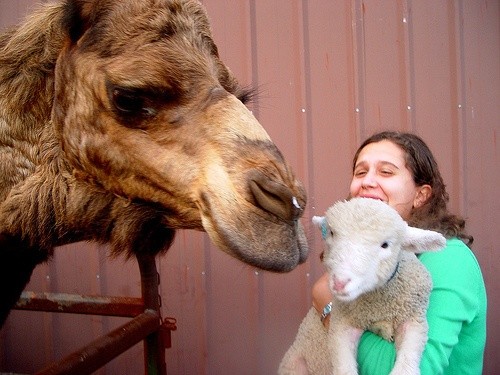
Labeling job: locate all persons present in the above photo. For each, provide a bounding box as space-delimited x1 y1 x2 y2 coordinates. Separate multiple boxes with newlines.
306 132 488 375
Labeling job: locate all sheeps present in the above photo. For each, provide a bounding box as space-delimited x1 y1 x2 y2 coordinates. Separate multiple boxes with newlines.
273 196 448 375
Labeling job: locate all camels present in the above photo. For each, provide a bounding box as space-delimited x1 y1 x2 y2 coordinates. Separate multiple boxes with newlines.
0 0 309 330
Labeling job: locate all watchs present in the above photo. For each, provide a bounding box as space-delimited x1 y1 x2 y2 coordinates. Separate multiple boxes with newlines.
318 300 332 326
